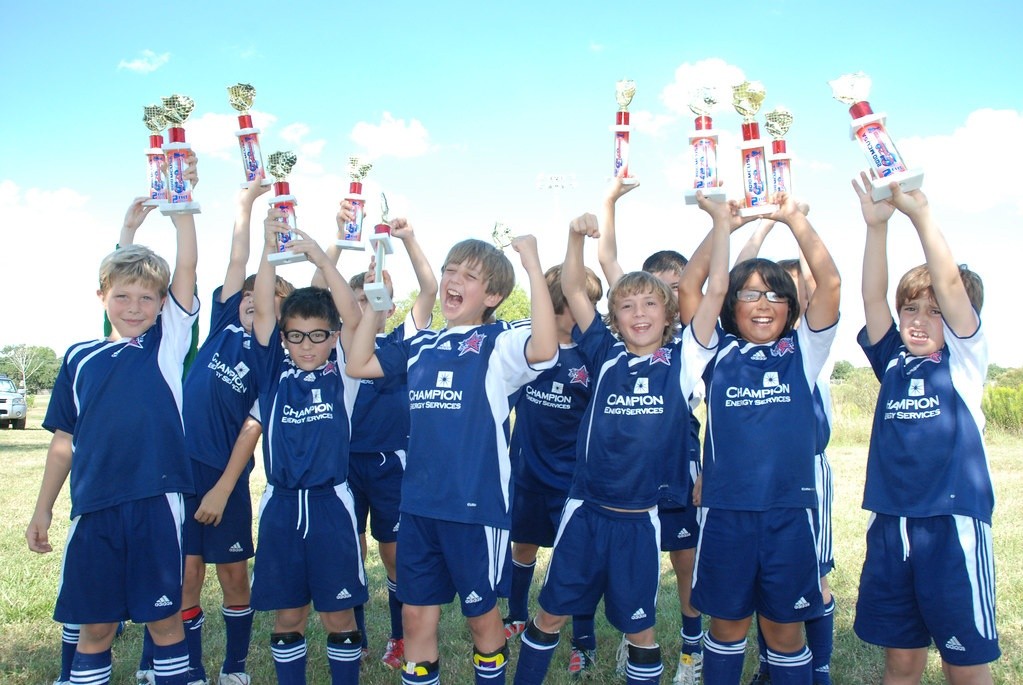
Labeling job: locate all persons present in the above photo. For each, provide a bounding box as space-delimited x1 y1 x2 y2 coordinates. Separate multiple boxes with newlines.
25 167 840 685
850 168 1001 685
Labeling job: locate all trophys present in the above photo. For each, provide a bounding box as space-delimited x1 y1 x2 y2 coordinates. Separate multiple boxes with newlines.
614 79 636 185
225 83 275 189
489 221 517 251
266 151 309 266
827 72 924 202
142 104 169 206
336 157 373 251
159 95 203 216
684 85 726 206
762 110 794 208
363 192 394 312
730 80 777 217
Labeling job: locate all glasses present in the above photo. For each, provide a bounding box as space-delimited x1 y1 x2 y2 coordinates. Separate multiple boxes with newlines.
736 289 789 303
283 329 335 344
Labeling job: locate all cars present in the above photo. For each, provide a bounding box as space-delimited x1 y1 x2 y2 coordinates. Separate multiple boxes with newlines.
18 385 25 396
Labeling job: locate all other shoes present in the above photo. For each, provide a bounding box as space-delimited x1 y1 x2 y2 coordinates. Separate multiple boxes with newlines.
749 673 769 685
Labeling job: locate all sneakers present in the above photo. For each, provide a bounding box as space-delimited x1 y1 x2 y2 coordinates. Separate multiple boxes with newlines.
188 678 210 685
570 638 596 681
673 649 703 685
135 670 155 685
217 666 250 685
615 634 629 681
501 615 529 641
381 638 405 671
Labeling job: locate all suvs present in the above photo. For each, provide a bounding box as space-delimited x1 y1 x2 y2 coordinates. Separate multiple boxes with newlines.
0 377 26 428
